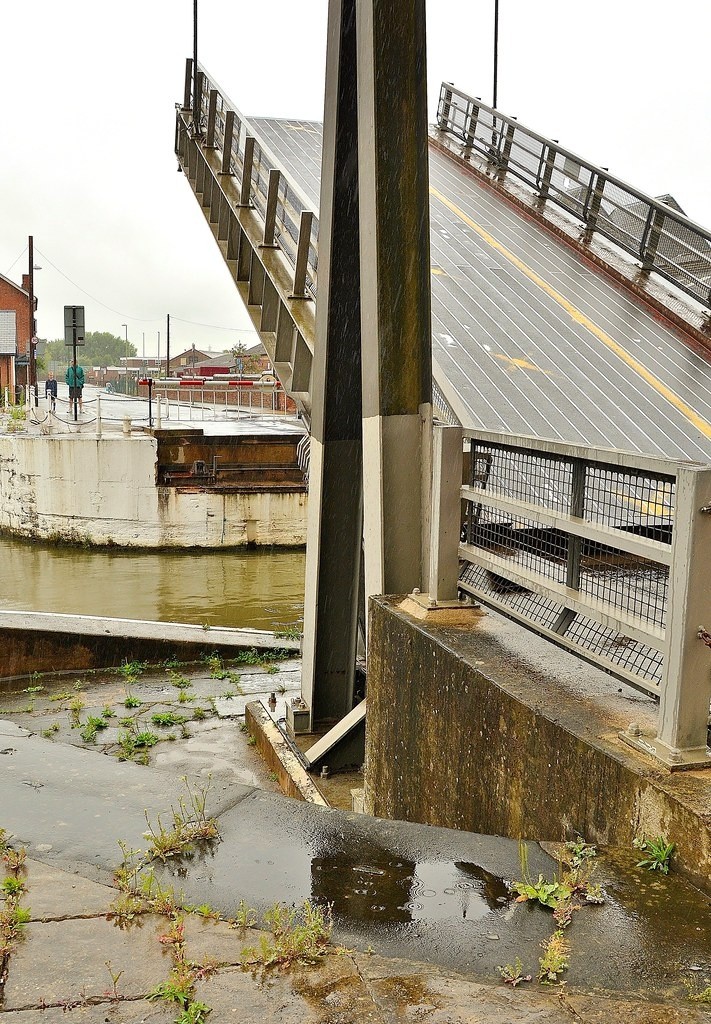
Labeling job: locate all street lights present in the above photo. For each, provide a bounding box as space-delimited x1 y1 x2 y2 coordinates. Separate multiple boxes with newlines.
122 324 128 395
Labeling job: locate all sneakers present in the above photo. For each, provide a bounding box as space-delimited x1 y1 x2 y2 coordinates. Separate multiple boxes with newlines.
80 409 85 413
67 409 73 413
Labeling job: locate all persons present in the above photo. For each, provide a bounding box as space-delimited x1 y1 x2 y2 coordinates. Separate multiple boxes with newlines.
65 357 86 413
45 371 57 413
106 381 117 394
